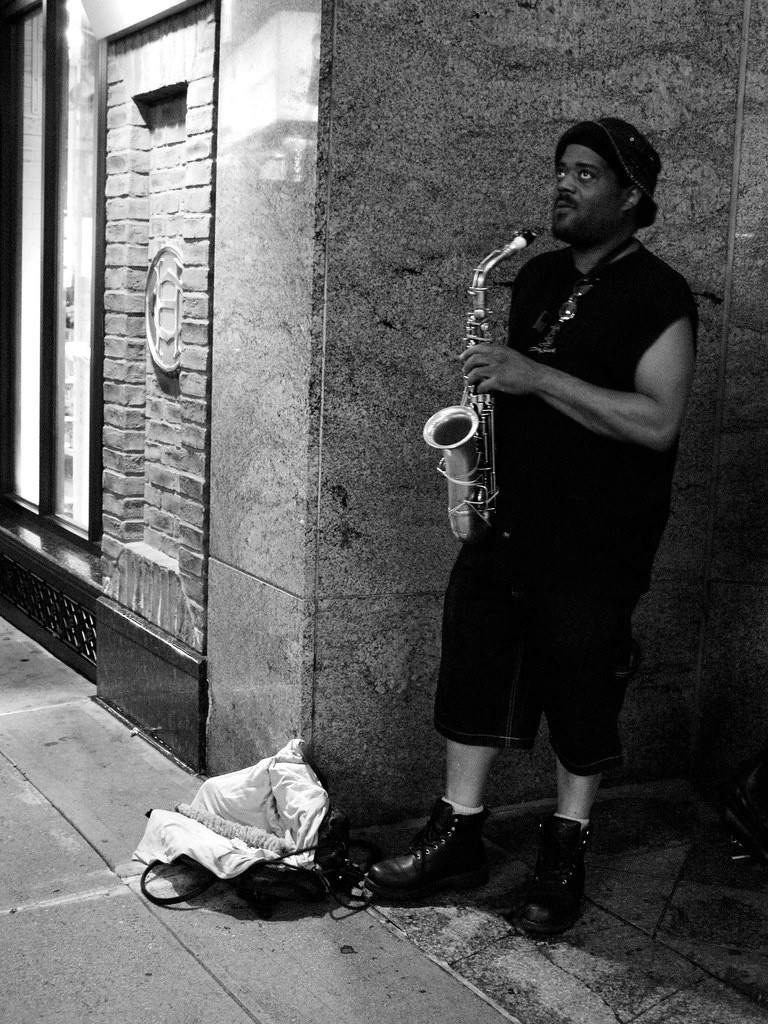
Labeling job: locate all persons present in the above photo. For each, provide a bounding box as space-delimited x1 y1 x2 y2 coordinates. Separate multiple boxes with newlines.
364 119 699 935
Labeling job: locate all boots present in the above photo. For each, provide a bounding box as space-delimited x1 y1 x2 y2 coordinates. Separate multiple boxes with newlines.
521 813 592 935
365 797 494 902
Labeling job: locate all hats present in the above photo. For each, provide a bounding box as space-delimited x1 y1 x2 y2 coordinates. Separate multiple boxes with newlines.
555 116 660 228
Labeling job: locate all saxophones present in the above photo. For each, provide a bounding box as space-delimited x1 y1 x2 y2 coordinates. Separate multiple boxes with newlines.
420 227 560 544
